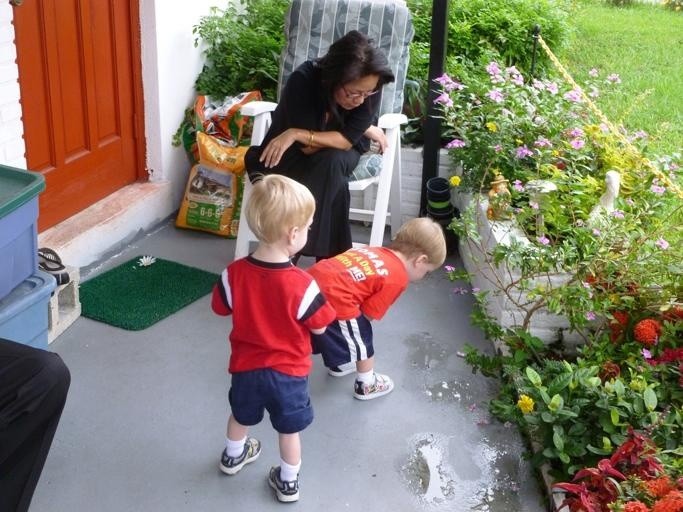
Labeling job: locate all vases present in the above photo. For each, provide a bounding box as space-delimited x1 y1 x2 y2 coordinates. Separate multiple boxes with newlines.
458 168 614 357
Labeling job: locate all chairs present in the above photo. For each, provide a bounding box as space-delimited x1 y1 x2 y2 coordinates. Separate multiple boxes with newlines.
233 0 415 261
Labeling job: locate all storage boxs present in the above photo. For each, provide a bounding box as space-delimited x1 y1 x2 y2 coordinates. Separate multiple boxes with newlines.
0 164 47 299
0 271 57 351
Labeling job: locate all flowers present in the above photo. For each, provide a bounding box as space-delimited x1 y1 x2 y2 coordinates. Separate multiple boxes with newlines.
428 59 683 256
137 254 157 267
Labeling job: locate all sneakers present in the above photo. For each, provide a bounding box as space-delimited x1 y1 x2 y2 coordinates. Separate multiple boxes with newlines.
328 362 358 376
220 437 262 475
356 372 394 400
268 464 300 502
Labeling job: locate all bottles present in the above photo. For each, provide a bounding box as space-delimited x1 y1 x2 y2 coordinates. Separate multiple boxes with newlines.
425 177 450 211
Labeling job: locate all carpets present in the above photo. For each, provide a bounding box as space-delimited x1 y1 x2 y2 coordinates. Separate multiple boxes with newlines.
79 255 223 331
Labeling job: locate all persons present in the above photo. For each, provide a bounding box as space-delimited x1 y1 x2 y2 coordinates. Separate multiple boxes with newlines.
302 216 449 403
208 172 337 504
244 29 398 266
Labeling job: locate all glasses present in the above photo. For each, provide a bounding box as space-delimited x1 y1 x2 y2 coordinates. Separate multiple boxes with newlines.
340 84 380 99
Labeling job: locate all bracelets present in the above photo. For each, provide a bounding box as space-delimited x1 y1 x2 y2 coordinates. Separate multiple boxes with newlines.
305 130 314 147
